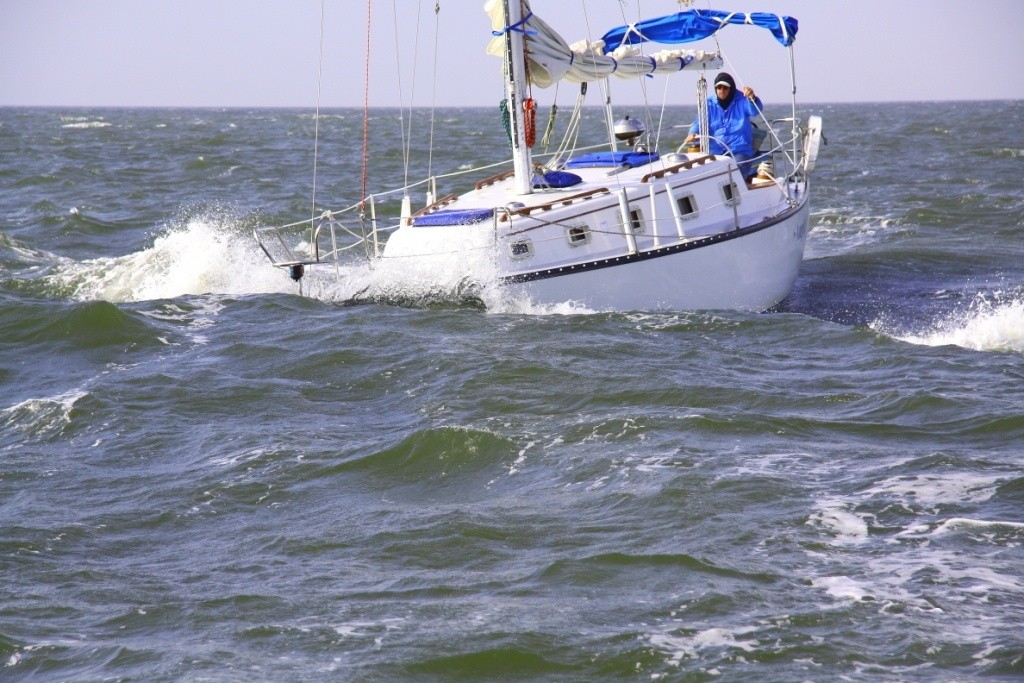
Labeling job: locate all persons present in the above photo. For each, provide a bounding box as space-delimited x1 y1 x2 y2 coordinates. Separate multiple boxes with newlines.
685 72 763 186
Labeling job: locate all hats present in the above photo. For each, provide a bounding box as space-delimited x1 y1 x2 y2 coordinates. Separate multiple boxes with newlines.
714 80 731 88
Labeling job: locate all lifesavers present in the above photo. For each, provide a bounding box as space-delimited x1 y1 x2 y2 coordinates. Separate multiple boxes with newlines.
803 114 823 172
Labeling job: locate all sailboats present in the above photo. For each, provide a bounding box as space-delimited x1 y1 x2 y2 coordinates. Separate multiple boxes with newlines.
253 0 831 316
